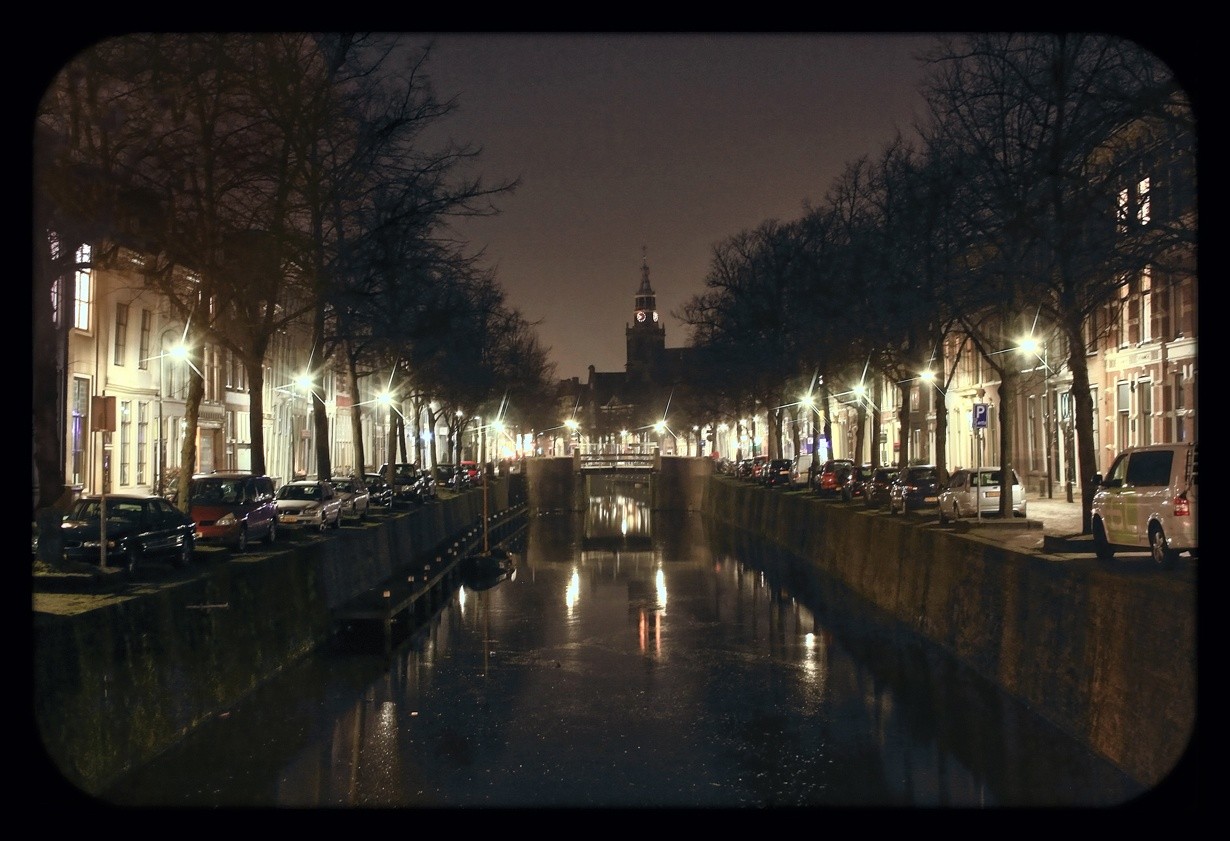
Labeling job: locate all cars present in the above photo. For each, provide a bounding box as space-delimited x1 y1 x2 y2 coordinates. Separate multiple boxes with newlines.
840 465 873 505
330 474 371 522
429 462 472 493
812 464 825 494
364 473 394 511
416 467 438 501
459 459 482 486
863 465 899 510
27 492 196 580
817 459 857 498
274 479 343 534
889 464 951 518
733 455 793 489
936 465 1027 527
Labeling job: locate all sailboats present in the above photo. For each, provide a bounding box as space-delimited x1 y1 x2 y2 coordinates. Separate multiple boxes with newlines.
460 430 516 589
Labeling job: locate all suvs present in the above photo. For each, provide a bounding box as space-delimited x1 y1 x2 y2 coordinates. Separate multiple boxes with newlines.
378 463 427 506
172 470 279 552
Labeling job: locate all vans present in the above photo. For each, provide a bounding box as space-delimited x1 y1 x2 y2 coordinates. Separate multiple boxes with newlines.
1088 441 1201 573
788 452 820 492
